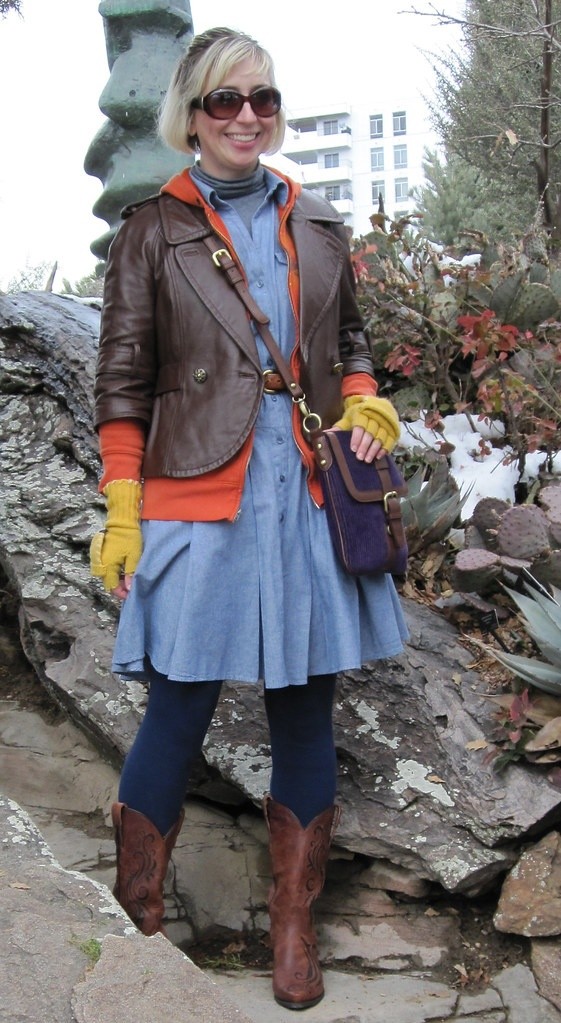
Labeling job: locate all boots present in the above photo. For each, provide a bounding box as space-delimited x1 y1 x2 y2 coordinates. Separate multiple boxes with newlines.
112 803 185 936
261 793 340 1010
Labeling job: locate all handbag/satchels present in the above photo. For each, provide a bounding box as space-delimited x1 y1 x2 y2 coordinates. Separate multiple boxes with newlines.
309 428 408 576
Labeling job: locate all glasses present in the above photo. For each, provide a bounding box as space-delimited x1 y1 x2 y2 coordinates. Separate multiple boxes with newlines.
191 86 282 120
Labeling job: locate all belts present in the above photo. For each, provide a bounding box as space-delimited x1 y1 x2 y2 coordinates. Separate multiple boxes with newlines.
261 369 287 395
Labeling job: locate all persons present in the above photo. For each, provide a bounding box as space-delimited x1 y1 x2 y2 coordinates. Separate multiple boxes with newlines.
88 26 400 1012
